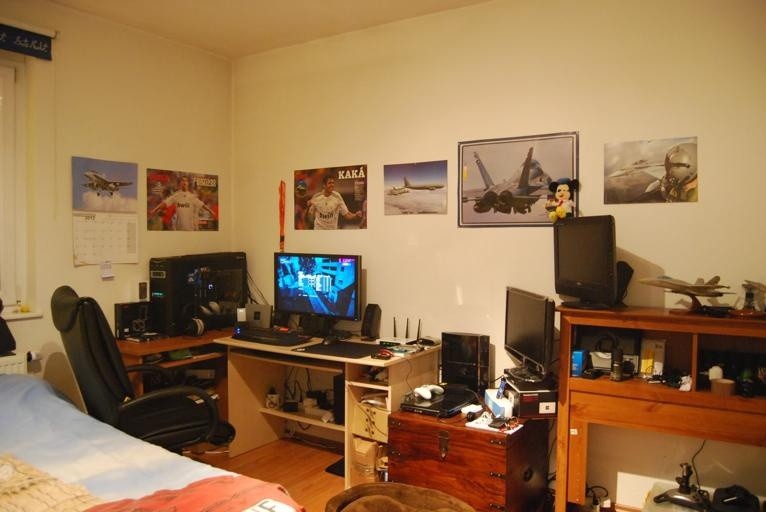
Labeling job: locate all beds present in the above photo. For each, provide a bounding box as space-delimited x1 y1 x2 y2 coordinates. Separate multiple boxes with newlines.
1 371 308 512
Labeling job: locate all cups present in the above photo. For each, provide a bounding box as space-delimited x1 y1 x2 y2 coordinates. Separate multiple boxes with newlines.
265 393 281 410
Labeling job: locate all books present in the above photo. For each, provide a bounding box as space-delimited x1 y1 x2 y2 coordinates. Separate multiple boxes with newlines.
389 345 419 355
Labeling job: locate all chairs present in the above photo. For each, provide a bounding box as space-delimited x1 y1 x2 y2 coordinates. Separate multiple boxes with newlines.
51 285 236 456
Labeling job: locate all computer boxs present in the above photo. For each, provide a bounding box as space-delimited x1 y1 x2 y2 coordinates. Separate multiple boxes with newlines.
149 251 247 336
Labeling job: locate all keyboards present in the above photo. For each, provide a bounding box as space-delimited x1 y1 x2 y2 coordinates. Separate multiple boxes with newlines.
232 328 307 346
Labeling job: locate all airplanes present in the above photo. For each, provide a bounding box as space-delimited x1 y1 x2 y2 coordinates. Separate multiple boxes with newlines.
610 158 666 179
386 186 409 196
461 144 555 218
391 174 445 193
77 171 133 198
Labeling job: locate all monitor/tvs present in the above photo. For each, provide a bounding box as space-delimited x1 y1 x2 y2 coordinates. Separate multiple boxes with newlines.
503 286 555 383
273 252 362 341
553 215 618 309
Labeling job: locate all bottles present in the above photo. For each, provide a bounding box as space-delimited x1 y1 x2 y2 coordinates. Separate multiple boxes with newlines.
744 293 754 310
611 362 622 382
739 366 753 396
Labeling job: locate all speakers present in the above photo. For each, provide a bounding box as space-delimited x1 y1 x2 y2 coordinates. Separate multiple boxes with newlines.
361 304 382 338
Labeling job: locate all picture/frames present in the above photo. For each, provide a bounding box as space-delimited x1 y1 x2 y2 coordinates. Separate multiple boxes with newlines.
457 131 578 228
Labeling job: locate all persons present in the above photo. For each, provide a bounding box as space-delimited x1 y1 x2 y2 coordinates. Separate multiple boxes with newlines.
660 142 697 202
295 169 367 230
147 174 217 231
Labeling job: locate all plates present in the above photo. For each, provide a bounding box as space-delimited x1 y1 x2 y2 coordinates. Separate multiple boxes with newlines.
728 309 765 318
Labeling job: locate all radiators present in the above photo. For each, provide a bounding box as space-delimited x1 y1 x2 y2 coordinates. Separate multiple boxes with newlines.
1 352 28 376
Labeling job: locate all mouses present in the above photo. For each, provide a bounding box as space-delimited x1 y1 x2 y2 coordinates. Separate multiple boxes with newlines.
371 349 392 359
323 336 339 346
